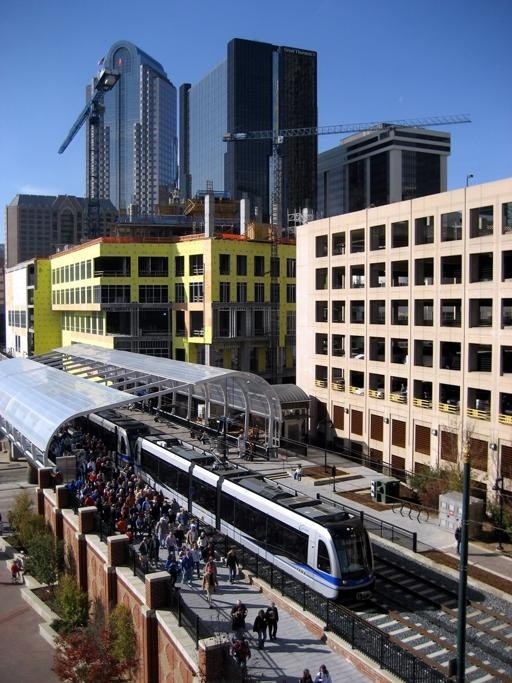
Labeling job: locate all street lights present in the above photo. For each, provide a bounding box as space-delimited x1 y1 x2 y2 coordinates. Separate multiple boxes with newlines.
316 419 333 473
493 475 504 551
217 417 227 462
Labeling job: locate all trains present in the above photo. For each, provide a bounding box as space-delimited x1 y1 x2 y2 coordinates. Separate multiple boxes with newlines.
67 409 376 601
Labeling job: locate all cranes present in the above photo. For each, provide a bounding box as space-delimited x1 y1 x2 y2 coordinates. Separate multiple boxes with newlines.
222 114 472 384
58 67 121 244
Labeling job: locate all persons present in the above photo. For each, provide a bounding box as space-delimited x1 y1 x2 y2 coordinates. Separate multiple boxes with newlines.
226 548 239 584
253 602 279 649
300 664 330 682
51 401 215 585
202 567 218 608
293 465 303 480
231 600 247 640
455 522 461 554
11 561 23 584
231 635 250 671
